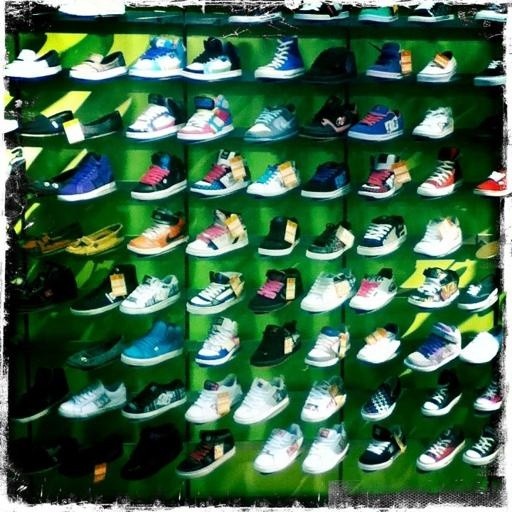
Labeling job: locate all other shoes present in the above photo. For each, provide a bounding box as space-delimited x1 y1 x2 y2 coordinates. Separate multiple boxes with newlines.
7 1 125 16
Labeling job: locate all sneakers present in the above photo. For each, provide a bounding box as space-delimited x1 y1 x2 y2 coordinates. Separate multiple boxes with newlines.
4 35 506 87
15 321 502 369
5 264 502 315
229 0 507 23
18 208 463 259
16 422 504 477
4 93 455 142
6 150 511 201
11 371 502 425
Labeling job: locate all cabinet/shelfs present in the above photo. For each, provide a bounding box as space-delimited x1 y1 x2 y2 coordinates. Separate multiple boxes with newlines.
4 18 506 495
